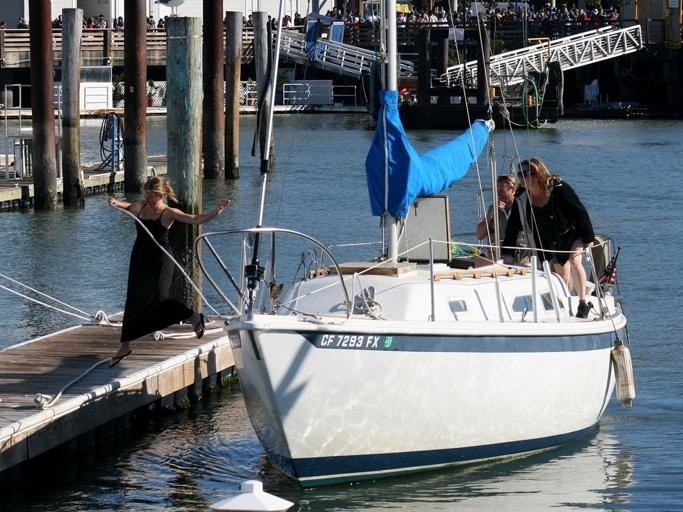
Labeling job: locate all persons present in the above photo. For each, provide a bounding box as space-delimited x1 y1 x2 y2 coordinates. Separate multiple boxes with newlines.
476 176 518 257
108 177 230 367
503 158 595 318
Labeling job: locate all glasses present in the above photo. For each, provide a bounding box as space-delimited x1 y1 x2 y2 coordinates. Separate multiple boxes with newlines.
517 171 530 179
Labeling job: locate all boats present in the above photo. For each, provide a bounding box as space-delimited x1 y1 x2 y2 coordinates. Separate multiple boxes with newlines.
398 69 548 131
191 0 627 490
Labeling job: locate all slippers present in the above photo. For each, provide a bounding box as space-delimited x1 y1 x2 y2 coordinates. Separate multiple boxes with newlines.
109 350 133 368
193 314 204 339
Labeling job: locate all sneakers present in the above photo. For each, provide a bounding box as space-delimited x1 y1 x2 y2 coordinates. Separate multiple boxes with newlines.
576 302 594 317
